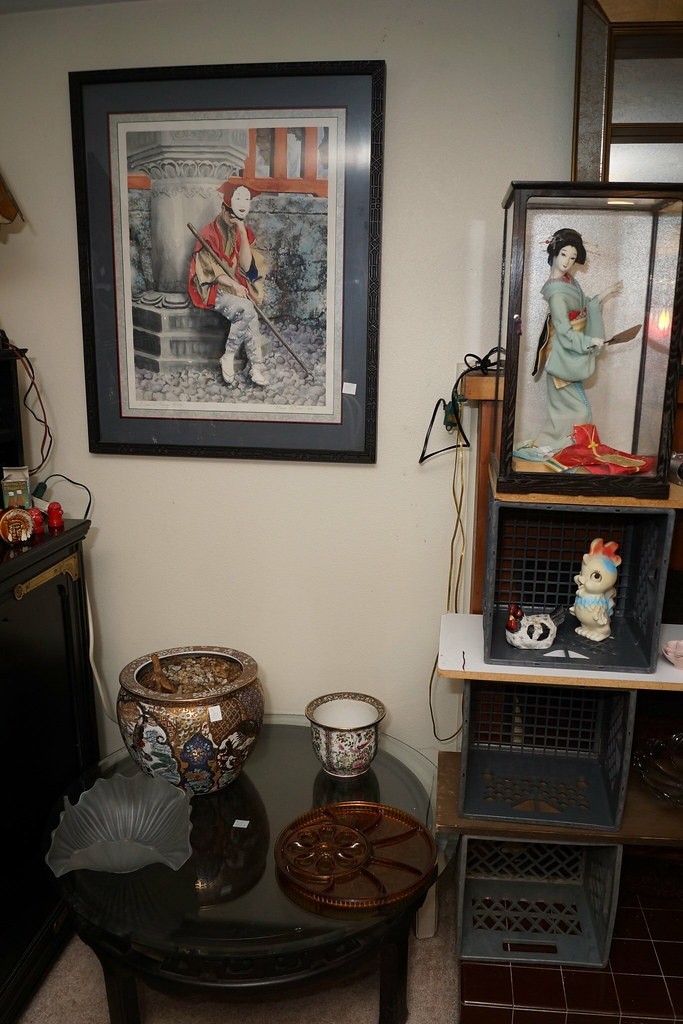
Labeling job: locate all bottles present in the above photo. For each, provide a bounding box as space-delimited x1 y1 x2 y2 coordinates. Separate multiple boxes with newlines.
184 774 271 908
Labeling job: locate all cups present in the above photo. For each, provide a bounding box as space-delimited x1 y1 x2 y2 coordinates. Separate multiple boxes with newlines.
305 692 387 778
310 769 382 808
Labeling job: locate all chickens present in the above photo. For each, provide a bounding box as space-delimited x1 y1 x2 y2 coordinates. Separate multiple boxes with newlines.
505 603 566 650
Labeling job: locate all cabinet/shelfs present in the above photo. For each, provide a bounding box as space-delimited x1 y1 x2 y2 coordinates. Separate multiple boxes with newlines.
0 350 100 1024
432 1 683 846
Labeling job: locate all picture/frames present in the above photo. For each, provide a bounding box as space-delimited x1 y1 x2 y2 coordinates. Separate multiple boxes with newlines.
68 60 387 463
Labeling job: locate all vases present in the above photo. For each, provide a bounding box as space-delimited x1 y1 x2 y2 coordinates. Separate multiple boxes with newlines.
305 692 384 778
116 645 267 797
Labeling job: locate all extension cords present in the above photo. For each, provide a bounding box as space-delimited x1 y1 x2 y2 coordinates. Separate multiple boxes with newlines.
31 494 52 515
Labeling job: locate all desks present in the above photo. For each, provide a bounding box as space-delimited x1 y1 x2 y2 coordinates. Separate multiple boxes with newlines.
61 712 459 1024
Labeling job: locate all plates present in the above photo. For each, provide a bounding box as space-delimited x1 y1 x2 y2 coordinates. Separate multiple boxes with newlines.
43 768 195 880
632 731 683 812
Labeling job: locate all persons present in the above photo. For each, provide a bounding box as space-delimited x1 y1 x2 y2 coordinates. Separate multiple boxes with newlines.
531 228 624 452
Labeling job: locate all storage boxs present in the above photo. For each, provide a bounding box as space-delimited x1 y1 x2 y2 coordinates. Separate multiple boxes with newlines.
483 484 675 675
460 679 637 830
456 832 624 970
2 466 32 511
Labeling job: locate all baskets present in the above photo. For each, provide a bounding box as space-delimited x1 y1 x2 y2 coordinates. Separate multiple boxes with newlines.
454 832 622 968
481 489 675 674
458 677 636 831
